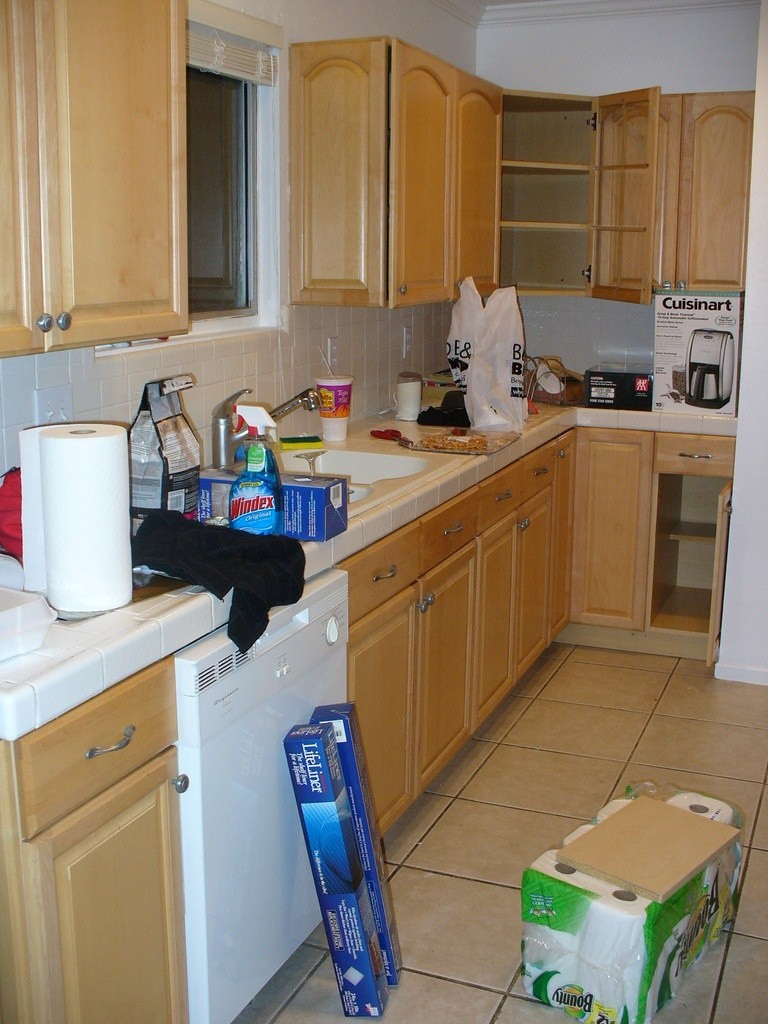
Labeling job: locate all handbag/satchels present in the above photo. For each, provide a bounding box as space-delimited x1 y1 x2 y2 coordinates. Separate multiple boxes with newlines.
523 352 585 406
446 275 528 433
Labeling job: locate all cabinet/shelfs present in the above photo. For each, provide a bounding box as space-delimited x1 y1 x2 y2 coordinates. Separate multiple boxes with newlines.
0 407 737 1024
0 0 189 358
292 36 758 308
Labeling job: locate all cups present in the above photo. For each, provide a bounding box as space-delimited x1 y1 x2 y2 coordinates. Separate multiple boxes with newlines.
314 374 355 442
394 371 422 421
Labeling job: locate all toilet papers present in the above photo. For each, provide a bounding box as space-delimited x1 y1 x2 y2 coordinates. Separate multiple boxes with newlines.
19 424 132 613
520 789 741 1024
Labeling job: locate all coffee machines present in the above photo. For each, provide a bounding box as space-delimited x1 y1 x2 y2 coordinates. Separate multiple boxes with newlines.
685 327 735 409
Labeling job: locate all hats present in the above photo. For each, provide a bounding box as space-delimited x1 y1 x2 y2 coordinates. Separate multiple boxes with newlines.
416 391 471 429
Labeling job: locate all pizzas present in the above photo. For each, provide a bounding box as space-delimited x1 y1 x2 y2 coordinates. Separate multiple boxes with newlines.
421 435 488 450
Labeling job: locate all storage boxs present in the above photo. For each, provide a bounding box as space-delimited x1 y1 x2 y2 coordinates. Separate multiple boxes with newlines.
654 290 742 420
198 467 347 542
284 723 389 1016
584 362 653 411
309 704 403 987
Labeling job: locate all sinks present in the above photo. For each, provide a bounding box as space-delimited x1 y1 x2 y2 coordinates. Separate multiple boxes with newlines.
349 484 373 506
229 447 428 485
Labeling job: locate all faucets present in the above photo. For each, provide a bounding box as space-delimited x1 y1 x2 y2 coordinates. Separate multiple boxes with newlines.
211 385 324 470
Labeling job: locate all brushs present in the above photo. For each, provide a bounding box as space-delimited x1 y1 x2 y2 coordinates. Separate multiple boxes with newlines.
538 356 584 382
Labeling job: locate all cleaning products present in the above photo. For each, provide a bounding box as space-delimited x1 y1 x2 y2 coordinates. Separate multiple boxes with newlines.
229 405 287 539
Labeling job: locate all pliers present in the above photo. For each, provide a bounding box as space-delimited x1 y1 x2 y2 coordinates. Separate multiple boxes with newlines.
371 429 414 448
